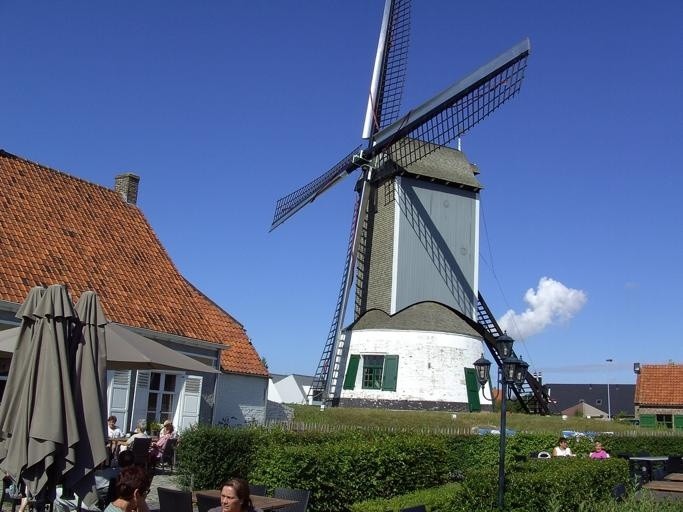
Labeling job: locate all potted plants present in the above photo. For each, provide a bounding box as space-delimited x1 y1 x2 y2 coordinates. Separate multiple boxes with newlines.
147 419 161 436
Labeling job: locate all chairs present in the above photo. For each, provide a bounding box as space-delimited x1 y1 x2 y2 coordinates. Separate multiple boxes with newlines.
2 476 21 512
154 438 177 475
125 437 153 475
248 485 267 496
157 488 193 512
196 493 221 512
270 487 310 512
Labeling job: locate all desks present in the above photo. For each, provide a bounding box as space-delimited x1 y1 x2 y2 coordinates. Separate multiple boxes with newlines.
196 488 300 512
101 434 159 468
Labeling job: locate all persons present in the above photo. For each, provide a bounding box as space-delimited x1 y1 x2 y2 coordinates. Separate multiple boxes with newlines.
107 416 178 468
552 437 572 456
590 439 607 459
207 478 264 512
103 465 149 512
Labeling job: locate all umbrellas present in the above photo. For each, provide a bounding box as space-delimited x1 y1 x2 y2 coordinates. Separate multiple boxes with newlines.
73 289 109 508
0 284 81 499
0 286 46 460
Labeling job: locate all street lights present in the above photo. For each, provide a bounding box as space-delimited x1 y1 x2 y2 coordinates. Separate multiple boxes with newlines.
471 329 529 508
